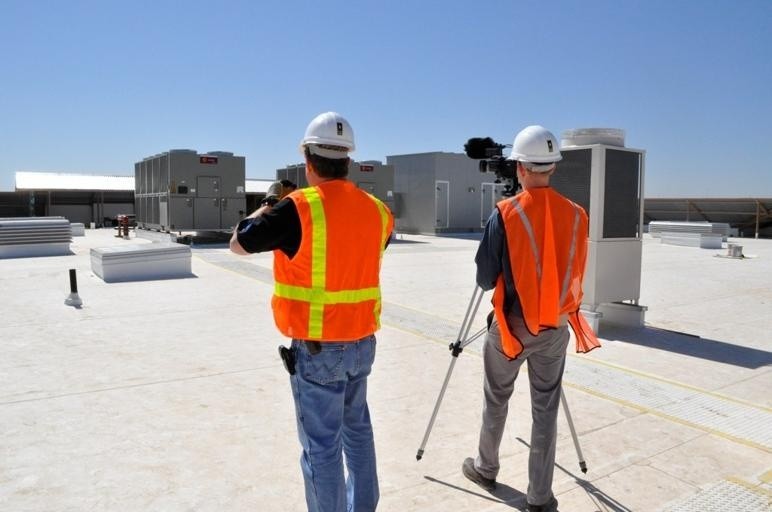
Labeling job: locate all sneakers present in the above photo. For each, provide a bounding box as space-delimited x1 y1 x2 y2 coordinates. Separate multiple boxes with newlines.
463 456 496 489
525 497 558 511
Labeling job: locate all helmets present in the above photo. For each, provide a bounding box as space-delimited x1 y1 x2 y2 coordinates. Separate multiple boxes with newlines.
505 126 563 163
299 110 354 154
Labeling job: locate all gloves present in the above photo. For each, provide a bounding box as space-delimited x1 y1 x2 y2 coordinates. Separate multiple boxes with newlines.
265 179 297 206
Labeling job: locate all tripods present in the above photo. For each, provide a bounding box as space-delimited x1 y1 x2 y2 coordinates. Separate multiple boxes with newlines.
414 193 589 473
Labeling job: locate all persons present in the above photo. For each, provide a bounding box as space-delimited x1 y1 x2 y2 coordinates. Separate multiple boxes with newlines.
458 124 600 512
228 110 395 512
